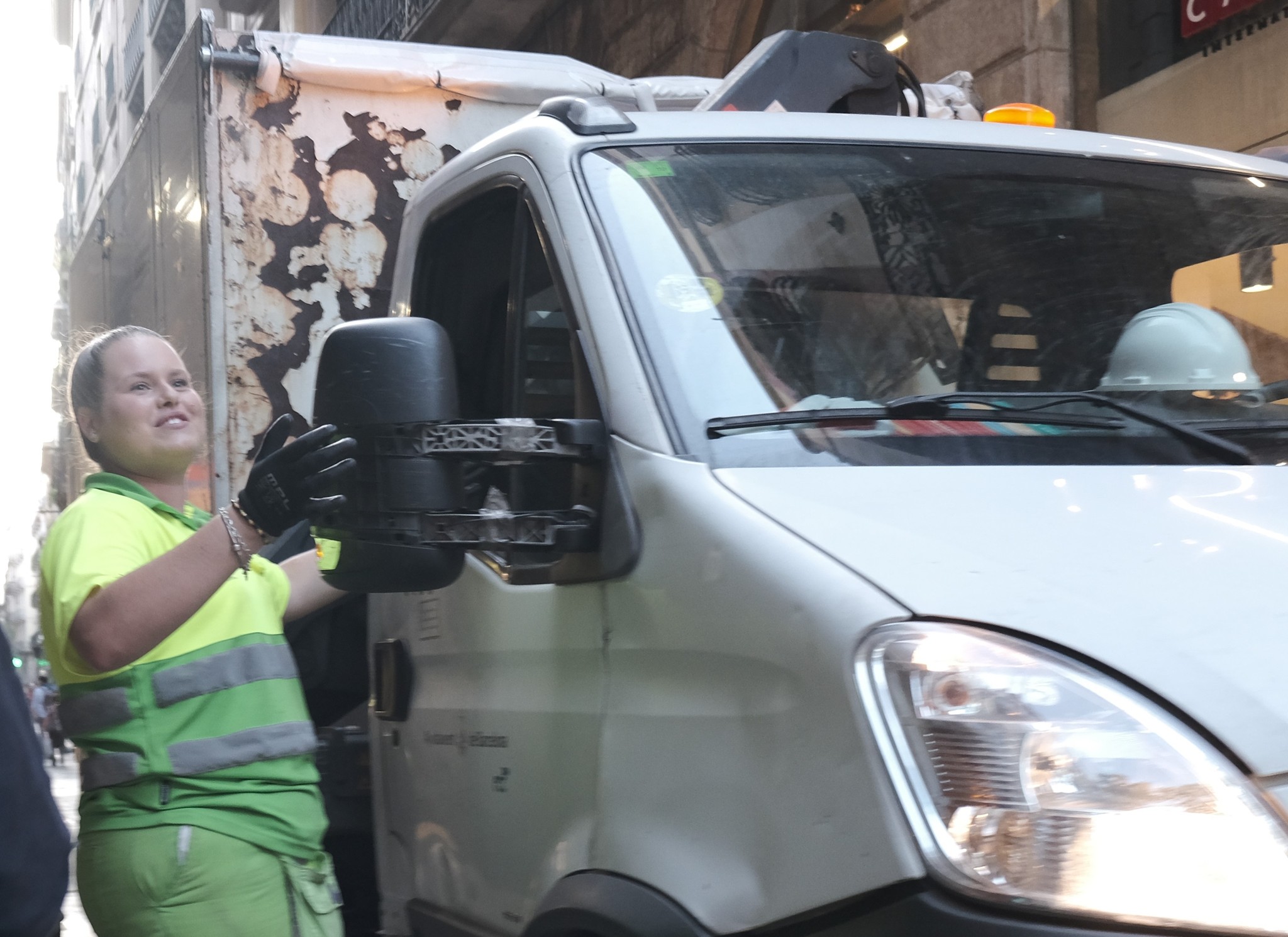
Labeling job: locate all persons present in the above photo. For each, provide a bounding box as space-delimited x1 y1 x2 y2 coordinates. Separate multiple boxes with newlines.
0 626 78 937
35 326 358 937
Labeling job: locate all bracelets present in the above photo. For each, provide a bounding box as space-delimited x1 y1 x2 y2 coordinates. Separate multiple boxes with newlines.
217 499 273 579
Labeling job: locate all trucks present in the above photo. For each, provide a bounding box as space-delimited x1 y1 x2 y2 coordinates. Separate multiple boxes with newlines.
49 4 1288 932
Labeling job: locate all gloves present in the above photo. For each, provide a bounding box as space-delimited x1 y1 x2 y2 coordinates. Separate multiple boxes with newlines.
238 413 357 538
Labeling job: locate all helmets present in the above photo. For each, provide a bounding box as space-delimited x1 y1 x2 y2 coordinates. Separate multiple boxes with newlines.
1093 303 1267 392
